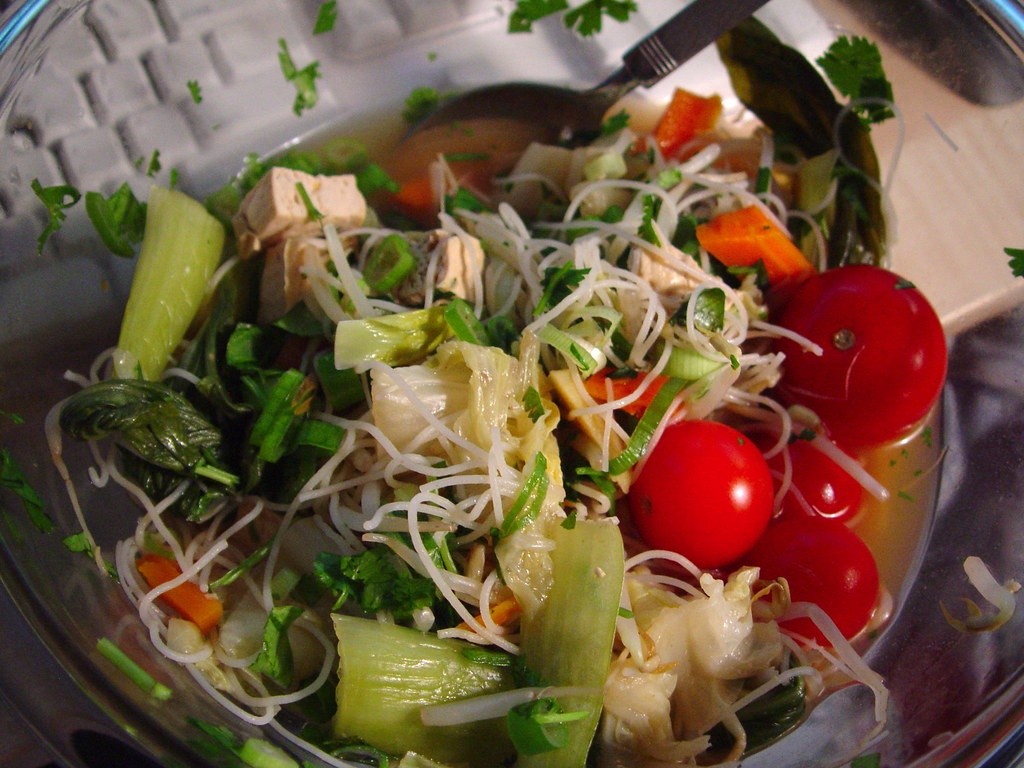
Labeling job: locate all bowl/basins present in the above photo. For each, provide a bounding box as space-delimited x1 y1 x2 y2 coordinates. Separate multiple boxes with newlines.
3 87 948 768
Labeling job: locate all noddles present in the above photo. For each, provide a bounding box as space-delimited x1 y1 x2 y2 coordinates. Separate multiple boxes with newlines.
73 90 948 766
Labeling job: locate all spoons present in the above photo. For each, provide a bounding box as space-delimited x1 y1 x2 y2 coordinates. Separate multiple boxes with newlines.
402 3 789 148
